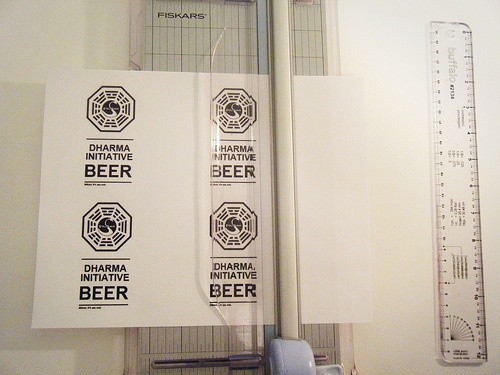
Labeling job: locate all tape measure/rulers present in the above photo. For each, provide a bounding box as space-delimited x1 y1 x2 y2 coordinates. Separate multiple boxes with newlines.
430 18 490 370
